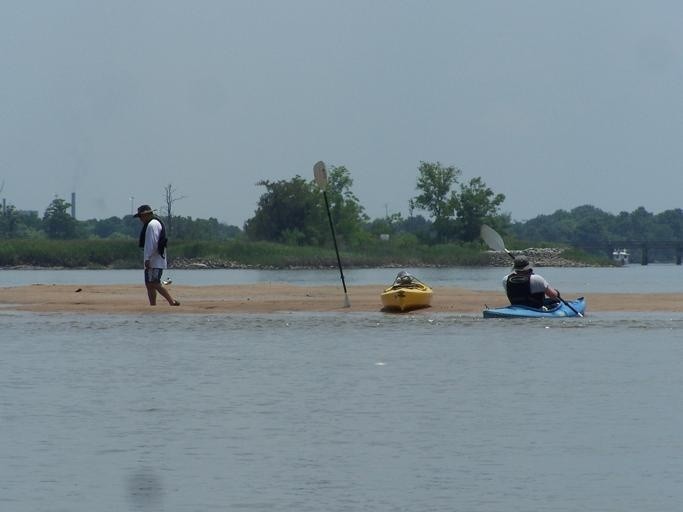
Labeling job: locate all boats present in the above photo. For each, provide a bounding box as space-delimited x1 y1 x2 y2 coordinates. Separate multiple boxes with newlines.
380 271 432 310
483 297 586 318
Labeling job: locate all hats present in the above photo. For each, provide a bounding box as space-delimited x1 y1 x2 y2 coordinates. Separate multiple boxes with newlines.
510 255 532 273
130 205 156 219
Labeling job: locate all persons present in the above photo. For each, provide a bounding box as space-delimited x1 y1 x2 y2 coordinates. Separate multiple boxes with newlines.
132 203 183 307
501 255 561 311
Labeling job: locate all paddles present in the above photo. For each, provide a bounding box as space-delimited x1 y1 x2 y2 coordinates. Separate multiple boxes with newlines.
313 161 349 308
480 224 583 318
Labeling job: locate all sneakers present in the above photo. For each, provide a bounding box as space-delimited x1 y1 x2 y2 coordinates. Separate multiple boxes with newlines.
170 300 179 306
